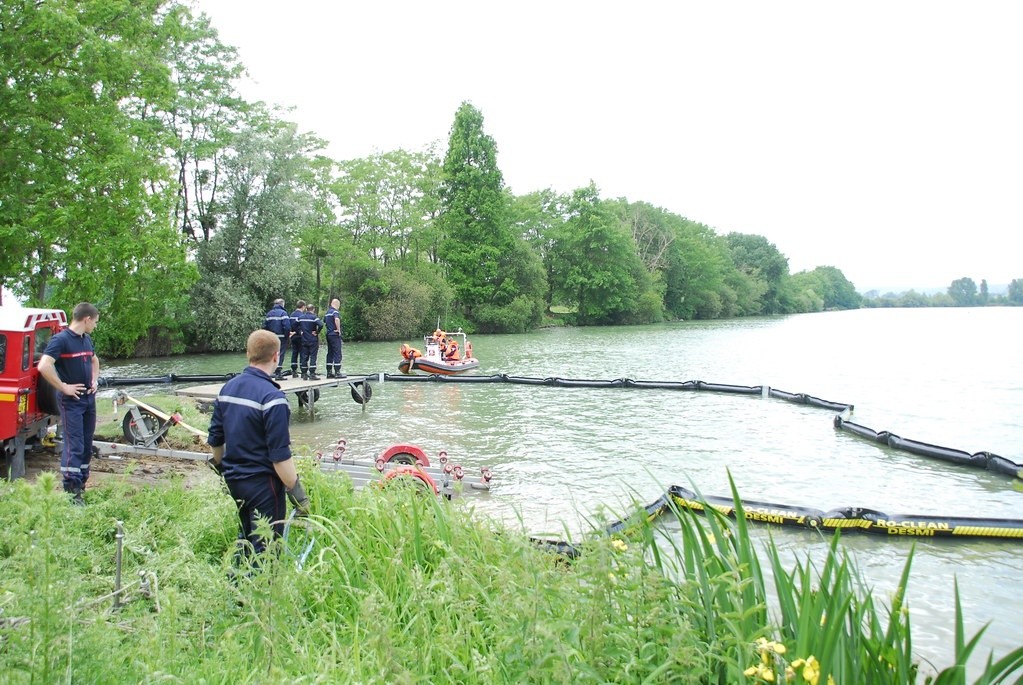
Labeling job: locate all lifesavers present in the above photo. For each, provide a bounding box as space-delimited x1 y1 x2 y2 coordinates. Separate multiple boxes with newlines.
350 381 372 404
301 388 320 404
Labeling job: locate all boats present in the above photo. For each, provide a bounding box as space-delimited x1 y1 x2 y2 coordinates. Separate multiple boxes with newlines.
398 318 479 375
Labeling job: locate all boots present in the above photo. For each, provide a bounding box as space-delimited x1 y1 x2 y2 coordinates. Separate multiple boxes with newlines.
335 370 347 378
301 369 309 380
310 370 320 380
327 369 334 378
63 479 87 506
292 368 299 378
275 370 287 380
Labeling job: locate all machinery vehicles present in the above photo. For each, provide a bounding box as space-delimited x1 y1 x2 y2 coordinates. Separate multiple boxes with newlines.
0 306 69 453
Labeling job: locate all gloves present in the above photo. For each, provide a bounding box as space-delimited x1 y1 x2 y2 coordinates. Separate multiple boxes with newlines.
208 458 230 495
284 474 311 521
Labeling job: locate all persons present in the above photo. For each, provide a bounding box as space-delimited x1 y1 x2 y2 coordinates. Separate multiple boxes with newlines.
36 303 100 508
294 304 324 381
322 298 348 379
432 329 472 362
398 344 422 373
207 329 311 609
261 298 291 380
289 301 307 379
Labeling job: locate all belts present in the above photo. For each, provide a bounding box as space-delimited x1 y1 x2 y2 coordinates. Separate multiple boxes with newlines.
76 389 92 396
